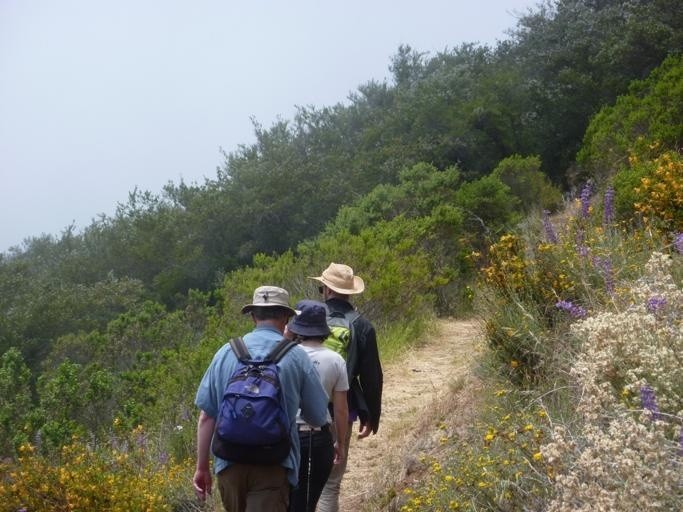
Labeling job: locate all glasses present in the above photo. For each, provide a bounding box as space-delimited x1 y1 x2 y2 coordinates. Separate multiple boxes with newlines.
318 285 325 293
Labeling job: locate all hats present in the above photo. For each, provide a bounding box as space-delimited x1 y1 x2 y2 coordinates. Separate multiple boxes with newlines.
287 300 331 337
308 263 365 294
242 286 298 316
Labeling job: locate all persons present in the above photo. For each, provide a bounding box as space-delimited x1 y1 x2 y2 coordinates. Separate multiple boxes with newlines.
286 299 350 511
192 285 331 510
310 262 383 511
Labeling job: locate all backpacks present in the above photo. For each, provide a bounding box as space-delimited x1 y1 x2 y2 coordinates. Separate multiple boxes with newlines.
323 311 361 401
210 337 299 463
